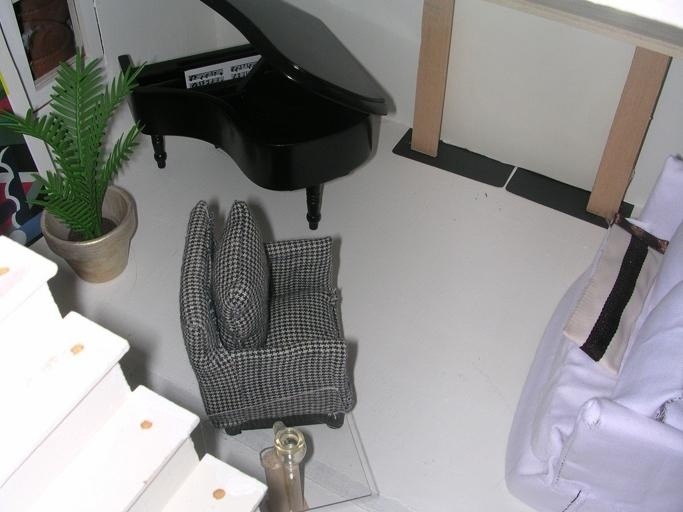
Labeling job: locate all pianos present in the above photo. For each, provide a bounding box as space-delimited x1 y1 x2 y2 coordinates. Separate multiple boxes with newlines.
118 1 389 231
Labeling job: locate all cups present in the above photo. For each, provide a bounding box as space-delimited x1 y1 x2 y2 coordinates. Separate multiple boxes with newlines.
273 421 308 468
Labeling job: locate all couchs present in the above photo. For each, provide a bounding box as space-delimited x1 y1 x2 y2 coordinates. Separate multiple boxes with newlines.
505 154 682 512
179 199 356 435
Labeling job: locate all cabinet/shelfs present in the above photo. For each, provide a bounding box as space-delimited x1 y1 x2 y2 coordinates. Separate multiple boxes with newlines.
2 0 117 248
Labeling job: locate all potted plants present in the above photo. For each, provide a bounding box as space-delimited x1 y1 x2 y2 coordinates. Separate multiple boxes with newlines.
0 44 148 284
